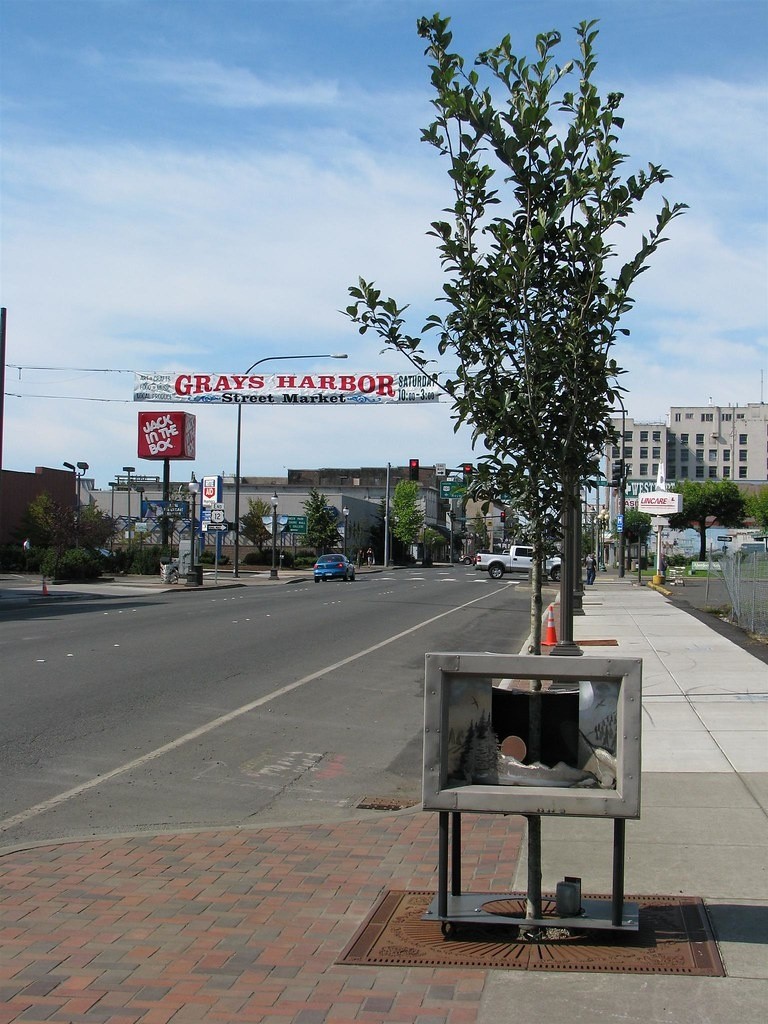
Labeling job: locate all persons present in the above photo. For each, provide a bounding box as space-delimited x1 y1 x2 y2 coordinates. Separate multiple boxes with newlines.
357 547 374 568
584 553 596 585
660 554 668 584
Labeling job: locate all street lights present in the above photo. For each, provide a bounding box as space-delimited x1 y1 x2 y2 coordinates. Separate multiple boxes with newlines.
231 353 348 578
63 461 89 548
270 490 280 580
342 506 350 555
108 482 118 551
597 509 611 572
185 471 200 587
122 466 146 550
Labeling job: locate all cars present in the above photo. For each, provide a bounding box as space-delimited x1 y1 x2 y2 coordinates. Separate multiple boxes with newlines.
313 553 355 583
502 549 510 555
94 548 112 558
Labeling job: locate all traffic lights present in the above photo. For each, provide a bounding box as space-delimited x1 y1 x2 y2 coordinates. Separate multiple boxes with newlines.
501 512 505 522
611 460 620 487
463 464 472 484
410 460 419 480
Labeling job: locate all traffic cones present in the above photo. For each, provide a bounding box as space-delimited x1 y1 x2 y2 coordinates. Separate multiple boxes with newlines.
39 582 51 595
540 606 560 646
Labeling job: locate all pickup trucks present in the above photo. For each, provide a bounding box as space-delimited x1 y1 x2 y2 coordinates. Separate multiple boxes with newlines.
459 545 562 582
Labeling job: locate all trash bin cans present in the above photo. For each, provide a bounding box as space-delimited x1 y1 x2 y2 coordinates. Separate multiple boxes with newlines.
188 565 203 585
631 559 638 572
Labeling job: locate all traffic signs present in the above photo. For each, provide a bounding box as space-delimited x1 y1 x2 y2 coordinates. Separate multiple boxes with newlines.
207 524 228 531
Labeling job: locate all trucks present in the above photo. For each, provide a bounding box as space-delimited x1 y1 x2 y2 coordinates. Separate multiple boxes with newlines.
741 542 766 564
